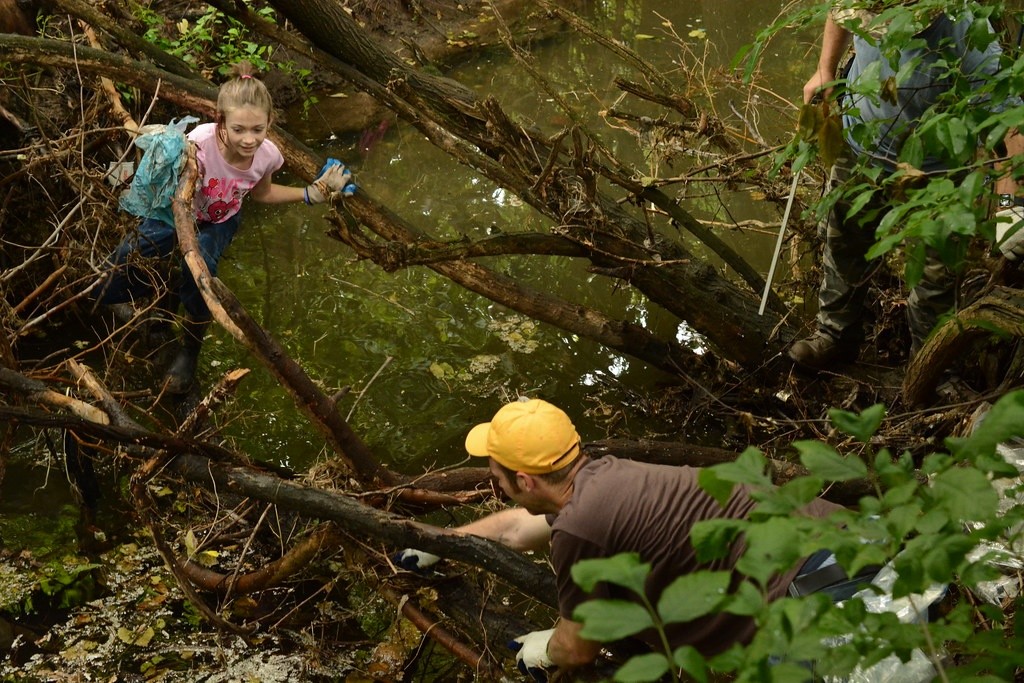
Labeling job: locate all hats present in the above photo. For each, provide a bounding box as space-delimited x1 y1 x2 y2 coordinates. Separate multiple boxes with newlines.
463 398 581 474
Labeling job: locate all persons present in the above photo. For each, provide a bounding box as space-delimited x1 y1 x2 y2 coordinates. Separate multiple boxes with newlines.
38 61 351 392
401 398 929 683
787 0 1024 378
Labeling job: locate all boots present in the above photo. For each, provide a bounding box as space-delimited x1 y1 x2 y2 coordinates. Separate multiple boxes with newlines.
162 310 211 394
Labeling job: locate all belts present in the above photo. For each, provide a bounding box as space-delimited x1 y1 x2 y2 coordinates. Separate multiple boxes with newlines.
793 559 884 597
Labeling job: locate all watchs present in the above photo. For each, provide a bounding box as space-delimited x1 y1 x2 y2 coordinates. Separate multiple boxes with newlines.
996 194 1024 208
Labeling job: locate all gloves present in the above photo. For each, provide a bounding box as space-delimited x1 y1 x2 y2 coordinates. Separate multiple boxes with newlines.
398 548 440 569
995 207 1024 260
304 157 356 205
508 628 559 683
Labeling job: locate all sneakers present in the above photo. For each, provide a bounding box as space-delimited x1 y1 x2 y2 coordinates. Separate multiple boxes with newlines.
791 329 851 364
